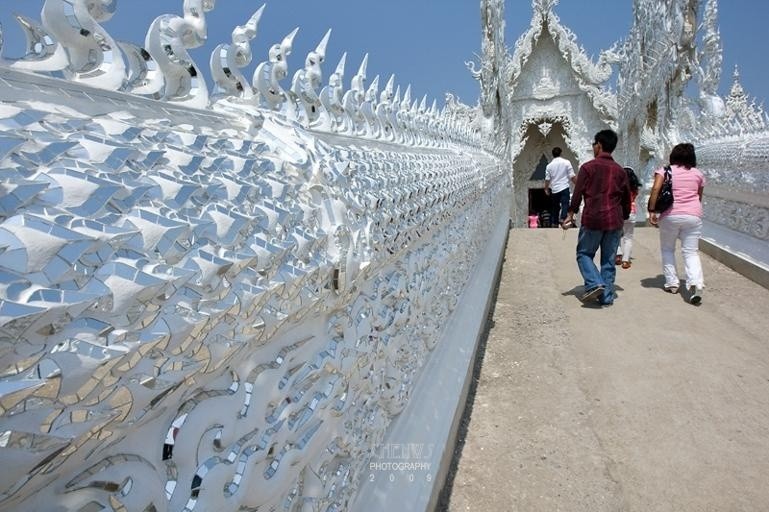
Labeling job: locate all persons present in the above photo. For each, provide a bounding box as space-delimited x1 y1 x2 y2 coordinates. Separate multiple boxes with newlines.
648 142 706 305
616 165 643 269
528 208 559 228
562 129 632 308
544 146 577 228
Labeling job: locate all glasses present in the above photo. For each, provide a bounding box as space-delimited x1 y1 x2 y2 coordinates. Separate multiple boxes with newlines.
592 142 599 147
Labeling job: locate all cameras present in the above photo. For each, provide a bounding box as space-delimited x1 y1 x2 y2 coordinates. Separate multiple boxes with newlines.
565 221 572 226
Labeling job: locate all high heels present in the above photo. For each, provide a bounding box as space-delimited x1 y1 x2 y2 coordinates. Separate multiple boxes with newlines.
689 285 701 304
663 285 680 294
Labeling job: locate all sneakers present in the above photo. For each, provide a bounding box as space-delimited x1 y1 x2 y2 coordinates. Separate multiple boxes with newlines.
550 224 559 228
582 286 604 302
616 255 622 264
599 300 613 306
622 262 630 268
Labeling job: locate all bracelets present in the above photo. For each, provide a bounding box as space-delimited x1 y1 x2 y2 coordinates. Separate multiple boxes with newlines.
649 209 656 213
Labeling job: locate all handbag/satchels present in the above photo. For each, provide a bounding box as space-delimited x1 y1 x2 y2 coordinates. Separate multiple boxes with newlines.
648 180 673 213
